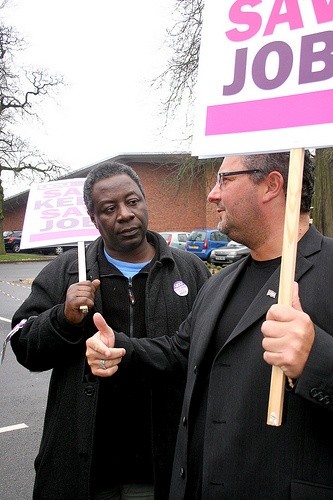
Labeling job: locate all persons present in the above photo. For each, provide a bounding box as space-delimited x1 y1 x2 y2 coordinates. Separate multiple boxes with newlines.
86 149 333 498
11 160 213 500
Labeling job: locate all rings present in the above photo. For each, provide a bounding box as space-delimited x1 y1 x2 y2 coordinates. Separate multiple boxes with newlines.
99 360 106 369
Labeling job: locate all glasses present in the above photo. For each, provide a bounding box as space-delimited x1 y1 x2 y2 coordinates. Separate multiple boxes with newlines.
217 169 260 185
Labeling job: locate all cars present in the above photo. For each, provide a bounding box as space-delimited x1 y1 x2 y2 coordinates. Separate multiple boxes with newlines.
1 228 264 265
